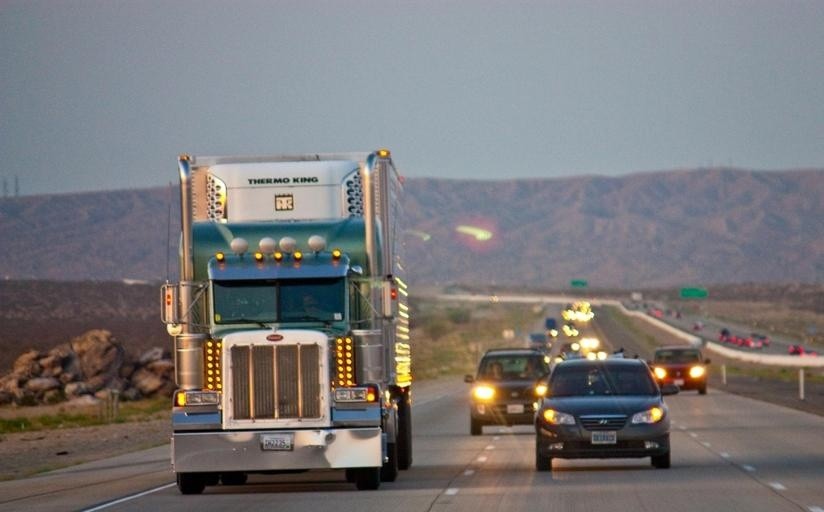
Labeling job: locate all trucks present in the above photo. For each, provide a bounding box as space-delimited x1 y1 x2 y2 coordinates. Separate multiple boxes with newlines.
158 148 413 495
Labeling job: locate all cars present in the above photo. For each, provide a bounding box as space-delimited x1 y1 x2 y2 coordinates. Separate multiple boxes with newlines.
717 329 818 357
